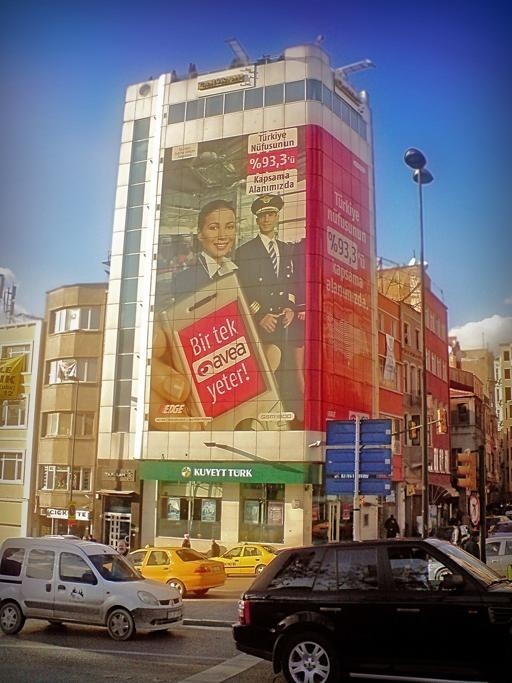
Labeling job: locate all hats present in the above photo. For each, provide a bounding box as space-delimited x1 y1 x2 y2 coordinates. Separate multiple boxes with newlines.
250 194 284 215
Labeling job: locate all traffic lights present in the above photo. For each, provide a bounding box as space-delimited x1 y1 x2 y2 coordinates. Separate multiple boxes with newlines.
456 451 477 489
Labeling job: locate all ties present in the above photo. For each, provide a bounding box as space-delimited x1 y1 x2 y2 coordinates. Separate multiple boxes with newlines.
267 240 278 279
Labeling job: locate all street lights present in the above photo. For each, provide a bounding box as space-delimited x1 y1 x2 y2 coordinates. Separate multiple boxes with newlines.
66 375 79 535
404 147 435 539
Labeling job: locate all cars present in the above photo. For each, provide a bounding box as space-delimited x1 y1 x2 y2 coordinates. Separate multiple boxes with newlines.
0 535 286 641
231 538 511 683
437 505 512 565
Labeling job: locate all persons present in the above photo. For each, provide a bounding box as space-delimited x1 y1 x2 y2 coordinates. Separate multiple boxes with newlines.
380 499 512 559
339 560 379 590
158 250 197 269
113 538 130 556
391 557 427 591
211 537 221 557
149 197 282 430
182 533 192 548
82 536 87 540
408 549 437 590
293 233 307 396
87 534 97 541
233 193 297 399
